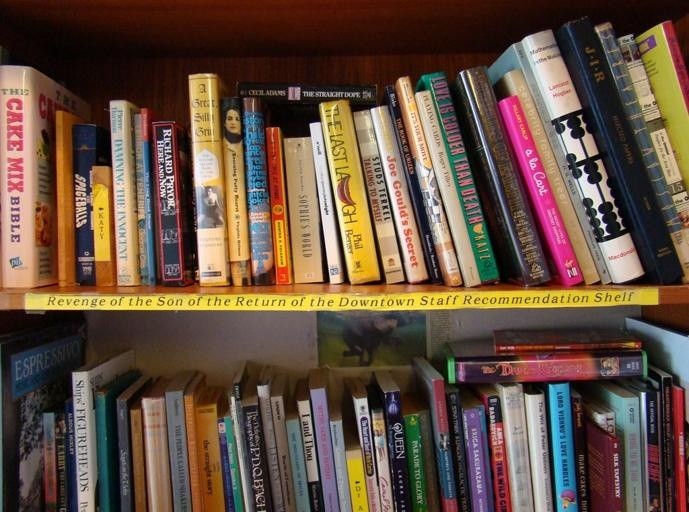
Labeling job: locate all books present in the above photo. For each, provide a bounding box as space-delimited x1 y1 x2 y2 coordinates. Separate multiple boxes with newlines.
585 420 625 512
618 35 689 239
446 385 471 512
0 65 86 290
270 375 297 512
222 105 252 287
373 370 412 512
88 166 115 288
108 99 145 288
318 100 381 283
56 414 68 512
51 110 87 286
307 371 339 512
166 368 198 512
229 394 254 512
488 44 613 285
226 414 244 512
282 139 324 284
628 381 664 512
327 406 352 512
456 71 515 284
131 113 156 290
188 73 231 288
42 410 59 512
72 348 135 512
400 413 428 512
416 408 443 512
555 18 684 287
477 386 512 512
497 383 534 512
117 375 153 511
217 419 233 512
412 357 458 512
65 400 77 512
594 22 689 283
265 125 293 285
371 410 395 512
673 385 687 511
447 342 649 382
130 405 145 512
258 364 285 512
524 29 644 285
598 383 643 512
395 76 462 289
153 121 193 285
144 377 172 511
571 392 592 512
244 387 267 512
493 326 640 351
461 404 487 512
383 87 441 282
348 381 381 511
286 416 311 512
415 90 481 288
620 384 650 511
238 81 376 105
636 22 689 192
506 69 600 287
97 367 142 512
597 403 616 435
585 401 606 430
546 382 578 512
10 334 82 512
233 358 245 432
345 440 369 512
473 400 496 512
296 381 324 512
371 103 428 283
500 96 583 286
648 368 675 512
241 99 273 286
310 122 344 285
70 124 107 286
199 387 227 512
184 372 207 511
468 67 551 288
351 110 405 283
420 73 498 284
523 389 554 512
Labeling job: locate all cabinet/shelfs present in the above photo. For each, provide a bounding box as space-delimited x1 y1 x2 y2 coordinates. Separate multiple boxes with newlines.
0 0 689 512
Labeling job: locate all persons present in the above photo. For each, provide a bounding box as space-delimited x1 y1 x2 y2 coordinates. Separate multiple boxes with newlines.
226 109 240 137
201 187 224 227
